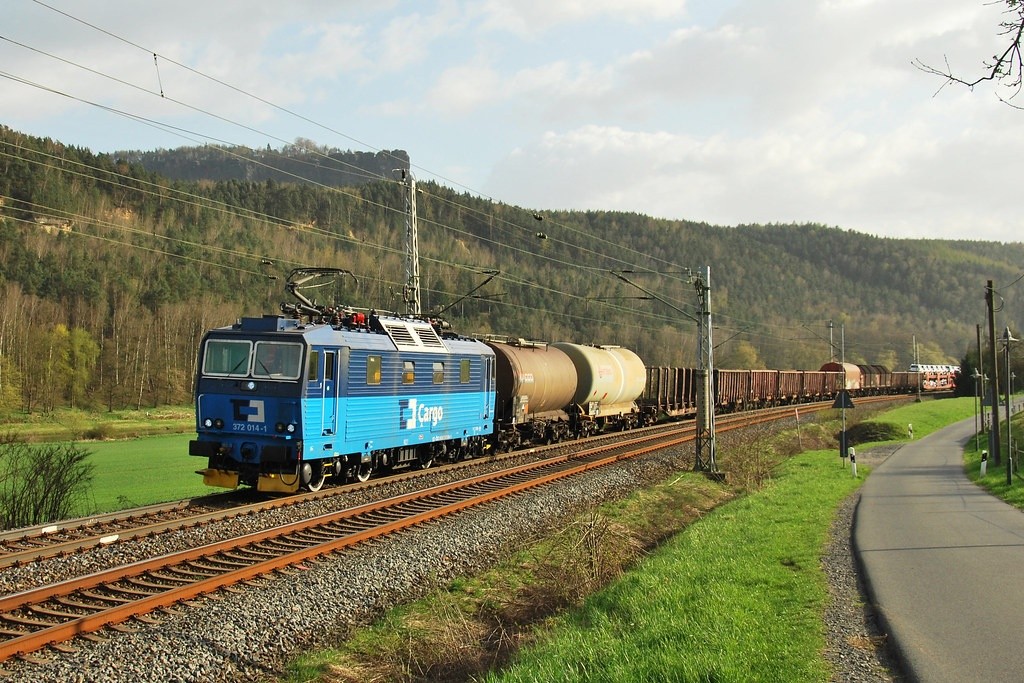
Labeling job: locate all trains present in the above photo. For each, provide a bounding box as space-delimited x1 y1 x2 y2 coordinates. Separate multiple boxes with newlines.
186 265 962 499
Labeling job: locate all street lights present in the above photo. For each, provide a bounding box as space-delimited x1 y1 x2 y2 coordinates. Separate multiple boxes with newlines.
1011 371 1016 415
969 368 982 451
983 373 989 433
997 326 1021 485
